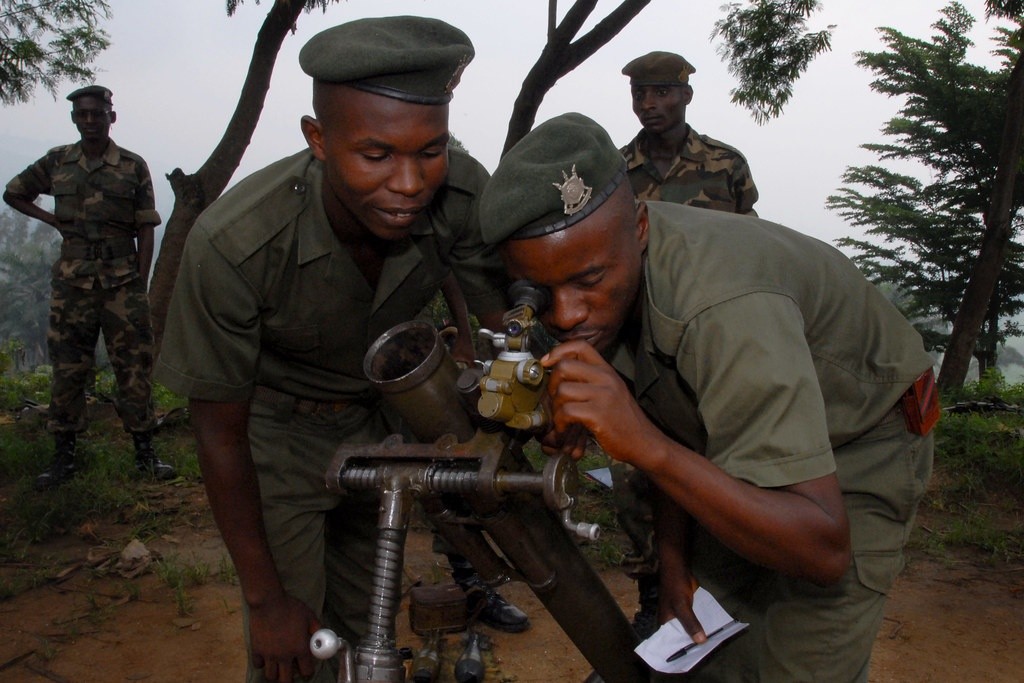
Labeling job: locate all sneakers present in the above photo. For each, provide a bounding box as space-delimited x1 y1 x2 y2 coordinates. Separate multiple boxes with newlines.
35 452 75 485
458 575 528 632
136 450 173 476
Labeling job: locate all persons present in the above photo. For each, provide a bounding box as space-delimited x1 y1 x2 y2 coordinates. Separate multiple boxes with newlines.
2 84 179 496
475 108 944 683
147 12 531 683
615 50 761 640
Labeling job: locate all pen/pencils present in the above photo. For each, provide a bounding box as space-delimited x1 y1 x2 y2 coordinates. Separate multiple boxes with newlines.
665 617 741 663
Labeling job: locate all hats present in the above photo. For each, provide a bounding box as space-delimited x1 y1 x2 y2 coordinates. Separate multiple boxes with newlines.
623 51 694 87
68 86 113 105
299 16 476 102
481 112 629 247
